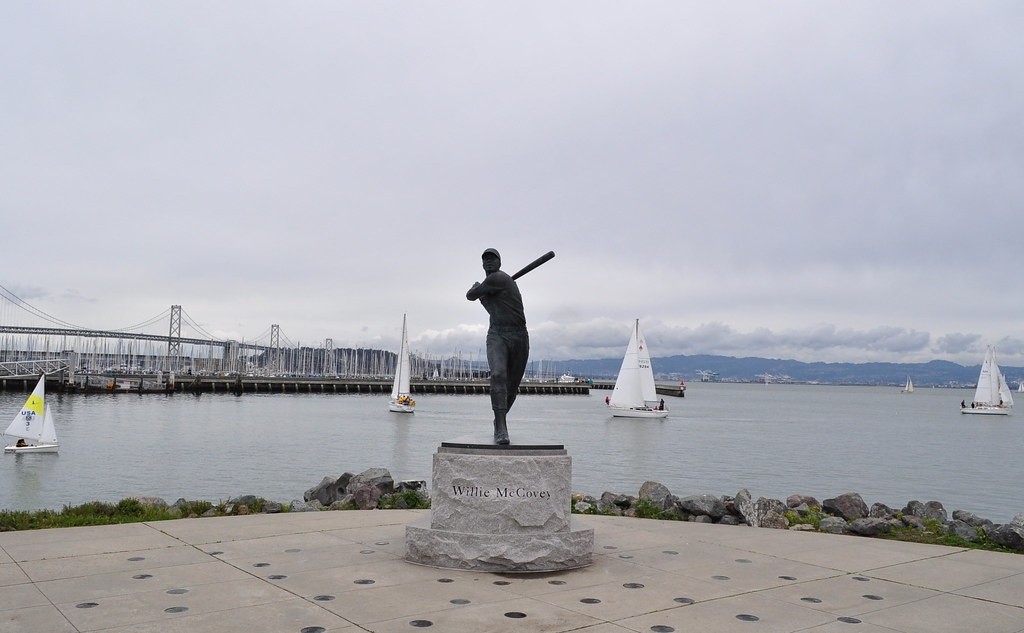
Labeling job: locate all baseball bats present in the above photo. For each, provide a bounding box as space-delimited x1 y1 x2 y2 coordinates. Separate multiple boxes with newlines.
510 250 556 282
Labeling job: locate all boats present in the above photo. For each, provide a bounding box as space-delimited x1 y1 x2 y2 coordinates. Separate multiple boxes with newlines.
80 375 166 392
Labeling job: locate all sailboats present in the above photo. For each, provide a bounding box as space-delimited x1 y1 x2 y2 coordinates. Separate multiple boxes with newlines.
388 313 416 412
605 318 671 418
2 374 60 452
960 345 1014 414
998 374 1012 395
900 375 914 394
1015 381 1024 393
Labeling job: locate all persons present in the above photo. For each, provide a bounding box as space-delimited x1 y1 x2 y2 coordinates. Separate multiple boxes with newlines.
961 400 965 408
659 398 664 410
466 248 530 445
